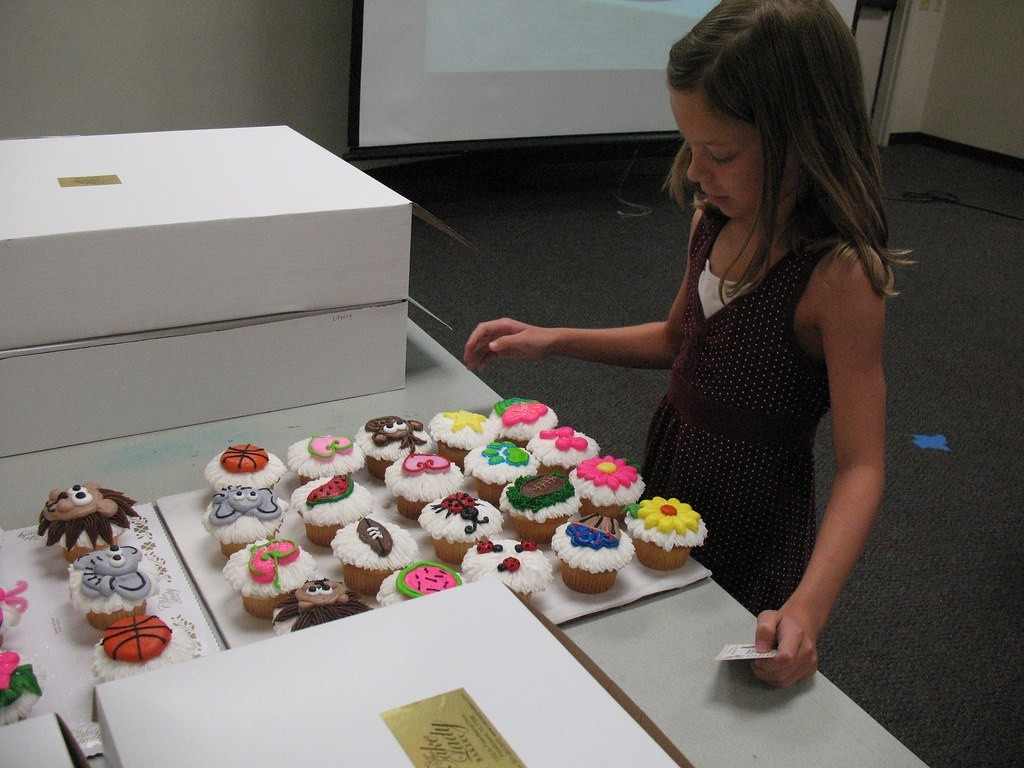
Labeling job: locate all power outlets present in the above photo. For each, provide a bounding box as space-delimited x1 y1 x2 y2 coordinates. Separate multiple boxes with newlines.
933 0 944 12
919 0 931 11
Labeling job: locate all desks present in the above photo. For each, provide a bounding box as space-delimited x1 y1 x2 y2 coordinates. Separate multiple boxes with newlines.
0 125 929 768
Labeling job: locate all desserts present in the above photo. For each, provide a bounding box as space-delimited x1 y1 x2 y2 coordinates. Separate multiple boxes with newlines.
201 397 709 637
38 481 193 682
0 579 44 729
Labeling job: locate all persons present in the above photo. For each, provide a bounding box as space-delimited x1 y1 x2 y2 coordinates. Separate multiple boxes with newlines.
464 0 919 689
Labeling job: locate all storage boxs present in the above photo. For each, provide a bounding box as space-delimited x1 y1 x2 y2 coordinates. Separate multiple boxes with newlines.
0 125 413 458
91 576 679 768
